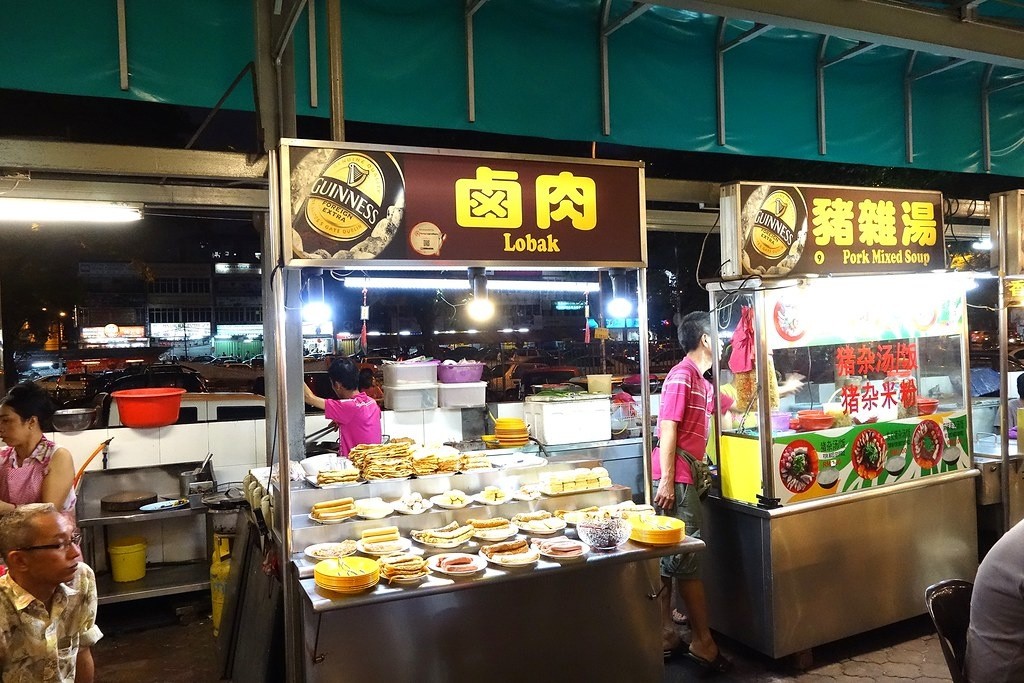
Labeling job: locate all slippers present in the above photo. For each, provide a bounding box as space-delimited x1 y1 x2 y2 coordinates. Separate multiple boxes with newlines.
664 639 690 660
687 649 734 674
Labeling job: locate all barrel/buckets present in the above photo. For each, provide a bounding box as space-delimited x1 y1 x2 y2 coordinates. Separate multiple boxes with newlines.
108 537 148 582
586 374 612 394
822 403 842 415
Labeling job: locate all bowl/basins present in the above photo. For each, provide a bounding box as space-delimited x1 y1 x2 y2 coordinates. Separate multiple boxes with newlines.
942 446 960 466
791 403 823 418
618 382 660 395
300 453 337 476
817 468 839 489
481 418 529 449
577 520 632 549
111 388 186 427
917 400 939 416
754 413 792 432
189 493 213 509
885 456 906 476
790 410 835 433
52 409 96 431
438 363 486 383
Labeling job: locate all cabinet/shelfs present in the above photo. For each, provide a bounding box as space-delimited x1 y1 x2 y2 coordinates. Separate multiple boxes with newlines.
75 459 219 607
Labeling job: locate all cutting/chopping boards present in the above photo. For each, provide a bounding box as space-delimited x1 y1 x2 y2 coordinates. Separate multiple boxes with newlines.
101 492 158 511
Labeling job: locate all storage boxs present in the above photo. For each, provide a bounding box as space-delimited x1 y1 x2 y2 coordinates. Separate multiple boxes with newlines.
437 380 487 411
522 398 612 446
381 383 439 413
380 363 440 386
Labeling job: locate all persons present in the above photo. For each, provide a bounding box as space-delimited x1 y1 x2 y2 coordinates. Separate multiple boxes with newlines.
303 357 382 455
993 372 1024 435
962 518 1024 683
649 309 807 678
705 368 759 467
0 381 84 575
358 368 385 403
0 502 105 683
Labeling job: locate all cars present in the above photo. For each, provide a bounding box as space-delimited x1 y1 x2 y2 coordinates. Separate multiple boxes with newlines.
15 353 339 425
345 340 713 403
968 330 1024 372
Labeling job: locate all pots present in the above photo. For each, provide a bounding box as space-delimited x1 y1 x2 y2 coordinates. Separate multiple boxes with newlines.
201 490 251 510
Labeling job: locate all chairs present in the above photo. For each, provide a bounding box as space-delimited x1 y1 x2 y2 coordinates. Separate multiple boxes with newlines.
924 579 974 683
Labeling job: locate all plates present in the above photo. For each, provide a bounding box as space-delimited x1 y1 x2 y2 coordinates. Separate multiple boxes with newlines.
426 539 590 576
412 523 518 548
140 500 190 512
357 537 429 584
538 485 613 497
392 491 541 514
774 301 807 341
518 505 655 535
852 429 887 480
304 543 380 594
780 440 819 494
912 420 944 469
308 503 394 524
368 476 411 484
305 476 366 490
459 467 500 474
415 472 457 479
627 516 686 547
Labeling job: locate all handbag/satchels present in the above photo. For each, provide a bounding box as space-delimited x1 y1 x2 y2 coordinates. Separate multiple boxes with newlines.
656 440 712 501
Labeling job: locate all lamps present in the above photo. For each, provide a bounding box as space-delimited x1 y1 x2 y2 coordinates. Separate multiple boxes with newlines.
466 266 494 323
0 197 146 224
302 267 327 325
607 267 633 319
646 210 720 234
944 224 991 244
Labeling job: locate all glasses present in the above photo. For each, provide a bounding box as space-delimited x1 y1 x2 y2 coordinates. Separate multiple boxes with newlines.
15 533 83 550
